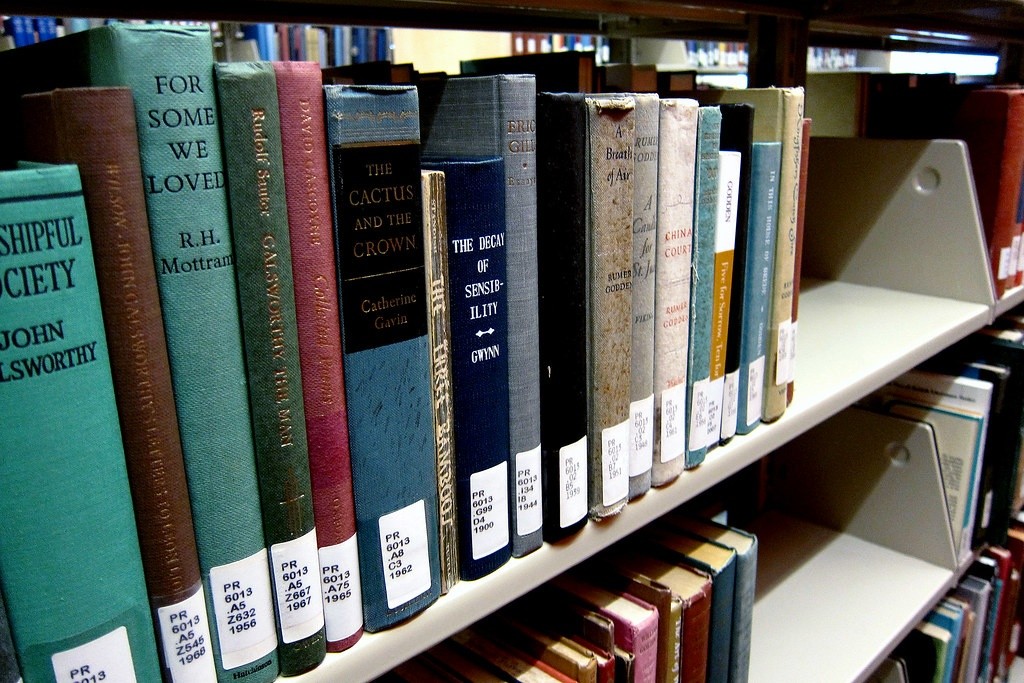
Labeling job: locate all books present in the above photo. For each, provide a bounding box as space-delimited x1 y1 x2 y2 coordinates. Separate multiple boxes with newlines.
0 21 1024 683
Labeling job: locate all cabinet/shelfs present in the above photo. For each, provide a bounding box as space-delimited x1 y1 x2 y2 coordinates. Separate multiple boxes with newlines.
0 1 1024 683
